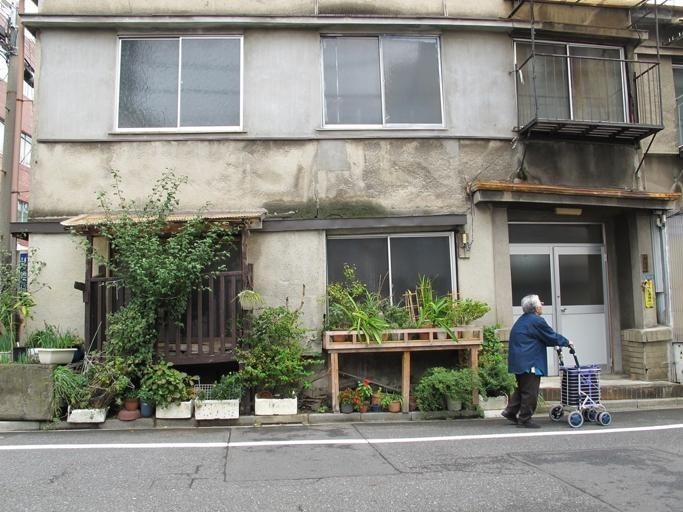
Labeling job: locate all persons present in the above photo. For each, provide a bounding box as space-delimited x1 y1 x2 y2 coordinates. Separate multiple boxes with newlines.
500 294 575 428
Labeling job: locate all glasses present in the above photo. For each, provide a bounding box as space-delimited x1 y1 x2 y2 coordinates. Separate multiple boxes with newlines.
540 302 544 306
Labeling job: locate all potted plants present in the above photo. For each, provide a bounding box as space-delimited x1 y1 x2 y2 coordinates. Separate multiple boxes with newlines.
416 367 477 411
389 308 404 340
84 369 130 410
415 277 436 340
337 388 354 413
69 166 237 386
382 393 404 413
52 368 88 410
34 323 79 365
353 311 384 343
478 328 503 369
477 365 515 410
403 319 419 340
327 264 364 330
460 299 490 339
141 364 193 418
354 380 372 413
370 388 383 412
426 299 448 339
139 387 155 418
373 309 390 341
0 238 49 323
239 303 320 414
326 305 347 341
194 371 238 419
124 391 138 410
447 303 462 339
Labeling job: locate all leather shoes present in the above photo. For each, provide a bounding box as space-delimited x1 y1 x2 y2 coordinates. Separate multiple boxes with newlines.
502 409 518 425
518 420 541 428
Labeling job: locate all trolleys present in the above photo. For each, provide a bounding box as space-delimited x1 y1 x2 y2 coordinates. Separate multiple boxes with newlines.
549 345 611 428
